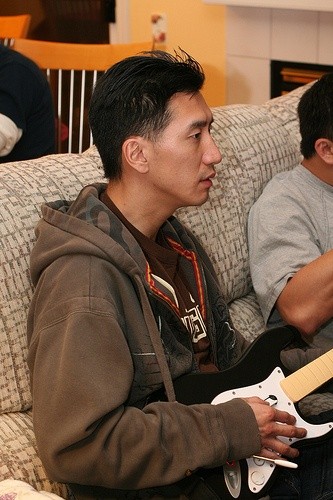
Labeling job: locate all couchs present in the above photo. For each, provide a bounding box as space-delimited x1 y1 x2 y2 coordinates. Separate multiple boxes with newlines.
0 80 333 500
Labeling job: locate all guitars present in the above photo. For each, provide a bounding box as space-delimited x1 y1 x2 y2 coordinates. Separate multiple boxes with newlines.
146 325 333 500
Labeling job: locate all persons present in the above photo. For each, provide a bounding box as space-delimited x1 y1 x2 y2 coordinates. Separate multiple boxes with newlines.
24 45 332 500
1 40 61 163
244 68 332 391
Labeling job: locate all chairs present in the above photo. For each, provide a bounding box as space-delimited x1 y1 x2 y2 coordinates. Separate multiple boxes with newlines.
0 14 34 48
12 38 156 155
